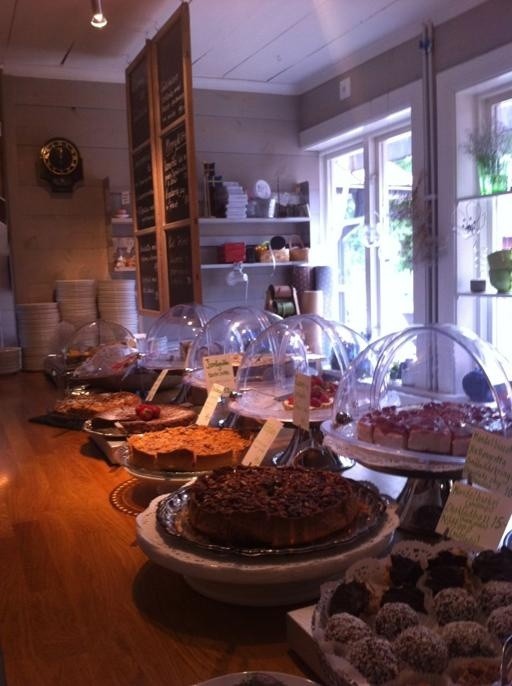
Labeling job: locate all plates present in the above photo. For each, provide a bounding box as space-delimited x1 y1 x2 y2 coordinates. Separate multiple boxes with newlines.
0 279 167 374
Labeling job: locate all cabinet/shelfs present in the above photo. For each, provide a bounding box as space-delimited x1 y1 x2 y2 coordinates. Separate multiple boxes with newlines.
104 174 139 271
197 174 312 274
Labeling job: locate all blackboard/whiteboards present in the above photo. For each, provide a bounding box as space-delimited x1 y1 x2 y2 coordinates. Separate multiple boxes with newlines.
126 2 203 323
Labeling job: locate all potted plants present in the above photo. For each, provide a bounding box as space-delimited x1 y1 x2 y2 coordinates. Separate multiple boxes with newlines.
457 113 510 195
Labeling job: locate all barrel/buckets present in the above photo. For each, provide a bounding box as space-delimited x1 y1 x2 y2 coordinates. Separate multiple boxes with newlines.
269 283 291 298
273 301 294 316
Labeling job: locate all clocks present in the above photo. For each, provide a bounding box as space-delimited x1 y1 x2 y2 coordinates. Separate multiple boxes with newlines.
37 136 85 194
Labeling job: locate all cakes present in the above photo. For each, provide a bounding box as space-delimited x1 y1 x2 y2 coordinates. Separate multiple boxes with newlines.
55 388 142 419
127 422 251 472
324 535 511 685
220 346 295 380
354 397 512 456
186 457 361 546
280 370 340 411
66 340 139 380
176 339 231 366
90 396 197 434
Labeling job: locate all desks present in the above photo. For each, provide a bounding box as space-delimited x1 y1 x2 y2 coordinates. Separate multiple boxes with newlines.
211 350 328 387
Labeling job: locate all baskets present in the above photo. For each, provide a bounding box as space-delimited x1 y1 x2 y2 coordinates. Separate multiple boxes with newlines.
218 242 246 263
290 247 309 261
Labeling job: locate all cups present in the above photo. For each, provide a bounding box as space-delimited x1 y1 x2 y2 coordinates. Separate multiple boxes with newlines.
488 249 511 295
